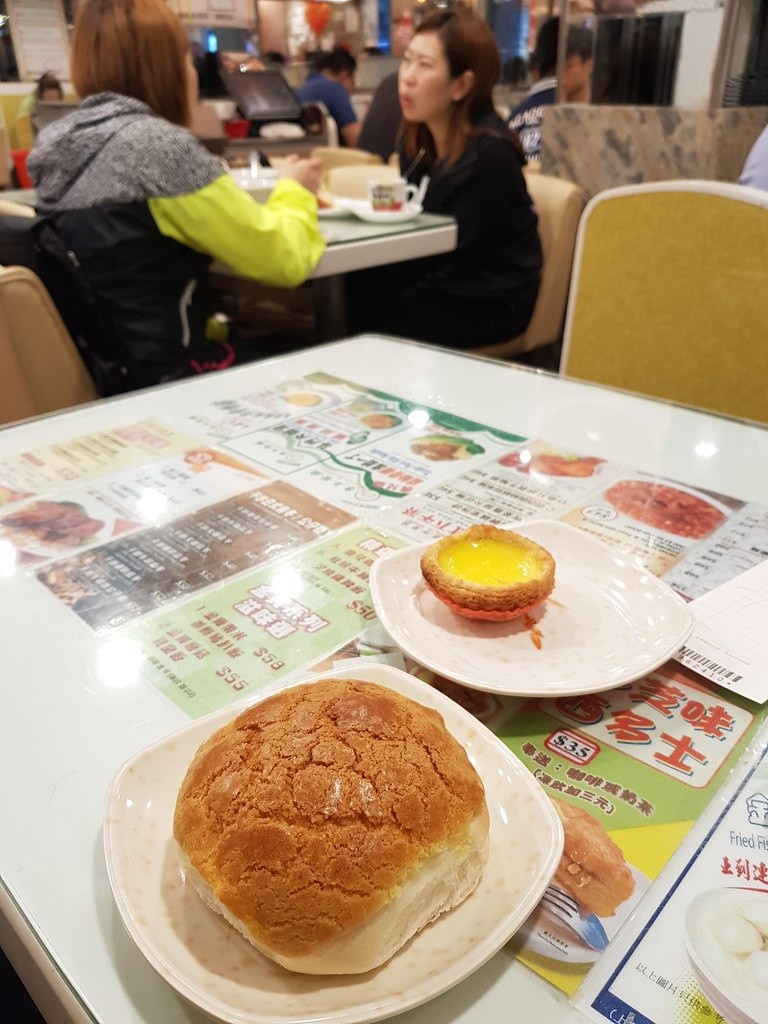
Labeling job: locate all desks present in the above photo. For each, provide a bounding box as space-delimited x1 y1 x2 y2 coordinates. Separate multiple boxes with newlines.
0 188 458 345
0 332 768 1024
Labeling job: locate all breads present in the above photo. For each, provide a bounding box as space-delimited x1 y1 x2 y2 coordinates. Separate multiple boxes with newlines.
172 679 490 974
549 795 635 917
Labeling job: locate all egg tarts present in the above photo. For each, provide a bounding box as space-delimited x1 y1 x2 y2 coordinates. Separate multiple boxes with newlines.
420 523 555 622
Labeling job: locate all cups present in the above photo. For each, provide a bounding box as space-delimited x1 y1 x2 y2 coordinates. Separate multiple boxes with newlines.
372 177 419 213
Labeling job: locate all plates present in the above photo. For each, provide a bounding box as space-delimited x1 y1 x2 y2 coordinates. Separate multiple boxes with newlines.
352 202 424 221
102 664 567 1024
515 862 651 962
369 520 696 699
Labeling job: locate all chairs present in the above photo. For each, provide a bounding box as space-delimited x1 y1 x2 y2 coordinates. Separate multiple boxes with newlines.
312 144 768 428
0 264 94 430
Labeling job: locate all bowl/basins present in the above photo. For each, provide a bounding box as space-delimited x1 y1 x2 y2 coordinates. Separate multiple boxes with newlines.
596 476 734 547
683 887 768 1023
238 179 276 203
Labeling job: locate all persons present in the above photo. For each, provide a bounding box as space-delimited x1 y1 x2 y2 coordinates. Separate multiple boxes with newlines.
297 49 362 148
739 124 768 192
19 75 62 116
316 11 543 344
503 16 594 168
34 0 323 381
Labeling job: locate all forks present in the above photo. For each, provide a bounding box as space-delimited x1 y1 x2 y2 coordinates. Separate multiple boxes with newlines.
539 883 609 949
